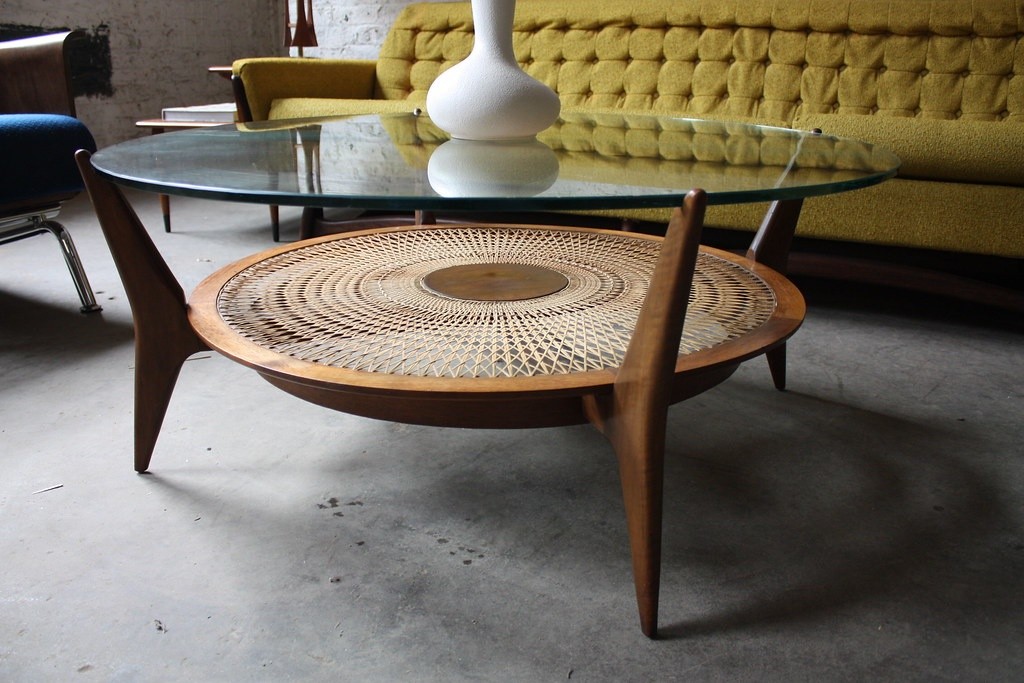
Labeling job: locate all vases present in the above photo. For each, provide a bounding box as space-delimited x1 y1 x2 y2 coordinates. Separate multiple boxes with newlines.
425 0 561 139
427 136 560 198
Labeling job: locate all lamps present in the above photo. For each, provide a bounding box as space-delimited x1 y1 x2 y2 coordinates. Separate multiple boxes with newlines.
284 0 318 57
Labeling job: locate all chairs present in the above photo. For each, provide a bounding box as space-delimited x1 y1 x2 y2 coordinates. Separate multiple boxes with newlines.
0 29 102 313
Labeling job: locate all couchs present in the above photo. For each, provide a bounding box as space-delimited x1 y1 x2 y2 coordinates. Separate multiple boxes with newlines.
230 1 1023 314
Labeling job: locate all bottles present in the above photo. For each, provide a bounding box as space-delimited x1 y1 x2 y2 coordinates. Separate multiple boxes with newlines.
426 0 561 141
426 135 558 197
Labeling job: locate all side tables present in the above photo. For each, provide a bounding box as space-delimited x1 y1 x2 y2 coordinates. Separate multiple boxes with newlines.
135 118 295 242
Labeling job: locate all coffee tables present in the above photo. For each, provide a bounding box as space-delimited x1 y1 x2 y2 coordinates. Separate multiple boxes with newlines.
75 109 903 638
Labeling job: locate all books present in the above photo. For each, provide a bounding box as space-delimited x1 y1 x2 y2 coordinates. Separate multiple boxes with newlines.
161 102 239 123
